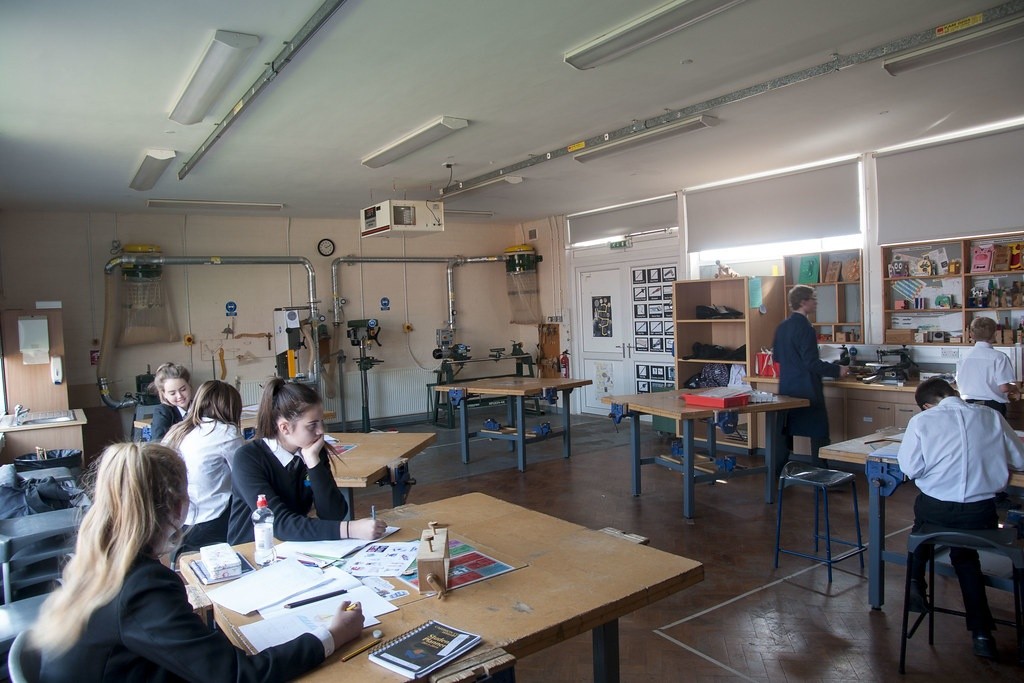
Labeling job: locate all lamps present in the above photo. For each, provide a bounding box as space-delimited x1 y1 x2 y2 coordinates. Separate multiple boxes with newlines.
147 200 283 211
564 0 749 72
169 29 260 125
128 150 176 191
882 16 1024 77
361 116 469 169
573 115 720 165
436 176 523 218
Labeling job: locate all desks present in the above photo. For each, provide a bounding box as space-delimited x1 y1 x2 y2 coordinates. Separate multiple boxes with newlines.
133 376 1024 683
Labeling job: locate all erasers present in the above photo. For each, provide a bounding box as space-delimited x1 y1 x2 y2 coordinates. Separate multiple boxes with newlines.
373 630 383 638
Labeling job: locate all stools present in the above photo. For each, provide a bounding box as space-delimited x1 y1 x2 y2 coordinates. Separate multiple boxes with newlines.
899 526 1024 672
775 461 867 583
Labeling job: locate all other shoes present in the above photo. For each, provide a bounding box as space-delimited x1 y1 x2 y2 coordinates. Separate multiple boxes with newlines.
974 637 997 657
993 499 1021 511
909 581 929 612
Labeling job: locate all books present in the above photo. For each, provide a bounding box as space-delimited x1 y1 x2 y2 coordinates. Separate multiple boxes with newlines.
368 620 482 680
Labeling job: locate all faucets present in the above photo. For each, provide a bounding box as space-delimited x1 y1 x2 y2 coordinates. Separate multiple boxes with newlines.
14 404 30 419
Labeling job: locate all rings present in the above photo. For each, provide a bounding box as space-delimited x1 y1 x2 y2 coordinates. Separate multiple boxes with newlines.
847 371 849 375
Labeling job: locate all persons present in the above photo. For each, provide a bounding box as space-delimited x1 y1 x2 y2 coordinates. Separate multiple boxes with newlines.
955 317 1021 422
772 286 850 488
227 378 388 546
153 363 194 445
897 377 1024 657
159 380 247 552
28 442 366 683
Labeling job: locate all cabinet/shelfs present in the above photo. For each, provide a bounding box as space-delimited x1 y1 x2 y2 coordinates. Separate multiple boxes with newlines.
0 309 88 470
672 230 1024 487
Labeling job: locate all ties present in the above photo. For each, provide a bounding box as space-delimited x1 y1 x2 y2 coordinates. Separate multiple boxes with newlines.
286 455 299 473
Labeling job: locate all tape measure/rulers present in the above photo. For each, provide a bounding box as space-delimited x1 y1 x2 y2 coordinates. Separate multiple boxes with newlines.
867 444 881 451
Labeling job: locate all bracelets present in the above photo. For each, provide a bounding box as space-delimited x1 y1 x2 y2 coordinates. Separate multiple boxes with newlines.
346 521 349 539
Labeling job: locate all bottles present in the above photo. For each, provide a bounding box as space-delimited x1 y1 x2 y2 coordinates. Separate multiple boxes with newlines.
849 329 856 342
252 494 277 567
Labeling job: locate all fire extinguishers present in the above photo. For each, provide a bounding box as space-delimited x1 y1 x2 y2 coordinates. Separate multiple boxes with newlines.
561 349 570 378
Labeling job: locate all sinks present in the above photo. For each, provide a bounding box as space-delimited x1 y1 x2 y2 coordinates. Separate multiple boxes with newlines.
21 415 71 426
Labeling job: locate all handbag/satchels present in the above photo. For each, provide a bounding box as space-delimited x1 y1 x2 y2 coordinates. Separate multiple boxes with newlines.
755 346 780 377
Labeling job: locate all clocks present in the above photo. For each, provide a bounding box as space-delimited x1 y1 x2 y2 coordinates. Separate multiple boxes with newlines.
318 239 335 256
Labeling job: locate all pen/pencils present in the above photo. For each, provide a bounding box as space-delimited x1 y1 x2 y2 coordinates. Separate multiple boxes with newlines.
277 556 315 564
372 506 375 519
345 604 357 611
342 639 382 661
284 590 347 609
273 560 318 567
864 440 884 444
13 449 82 487
